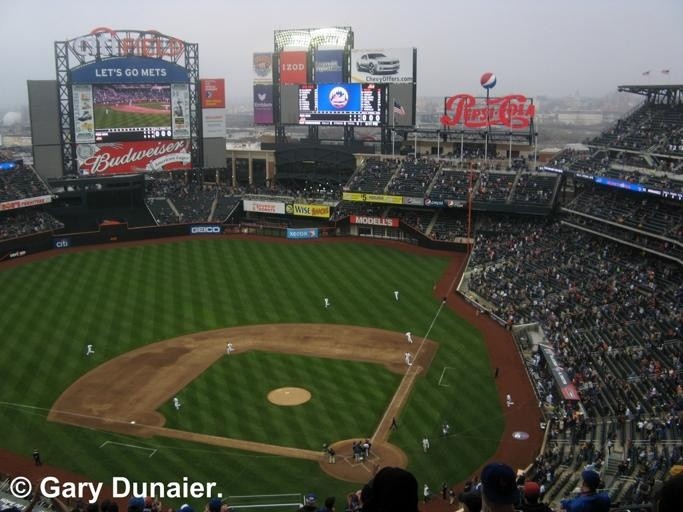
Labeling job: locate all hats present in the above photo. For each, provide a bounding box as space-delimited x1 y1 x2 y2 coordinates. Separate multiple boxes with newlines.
583 469 600 487
359 466 417 512
209 493 230 510
522 481 539 493
177 504 193 512
127 495 153 512
480 464 517 501
670 465 683 476
306 493 316 503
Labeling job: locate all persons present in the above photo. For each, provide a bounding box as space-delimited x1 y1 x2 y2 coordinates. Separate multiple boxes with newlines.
0 428 457 511
458 94 682 510
388 416 398 430
403 351 413 366
85 343 94 357
171 395 180 411
0 149 566 246
226 341 235 355
324 296 329 308
403 330 413 344
441 295 446 304
391 288 399 303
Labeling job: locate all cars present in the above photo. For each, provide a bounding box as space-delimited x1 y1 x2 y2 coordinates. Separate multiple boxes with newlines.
357 53 401 74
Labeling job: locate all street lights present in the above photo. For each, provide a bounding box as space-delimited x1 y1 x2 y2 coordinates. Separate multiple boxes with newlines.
534 132 539 173
483 131 488 166
390 126 396 158
437 129 441 162
460 129 465 164
413 129 418 159
508 131 513 170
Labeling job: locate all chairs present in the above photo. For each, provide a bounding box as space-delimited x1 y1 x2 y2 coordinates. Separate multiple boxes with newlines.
0 163 63 246
346 146 564 253
141 171 339 241
541 99 683 280
